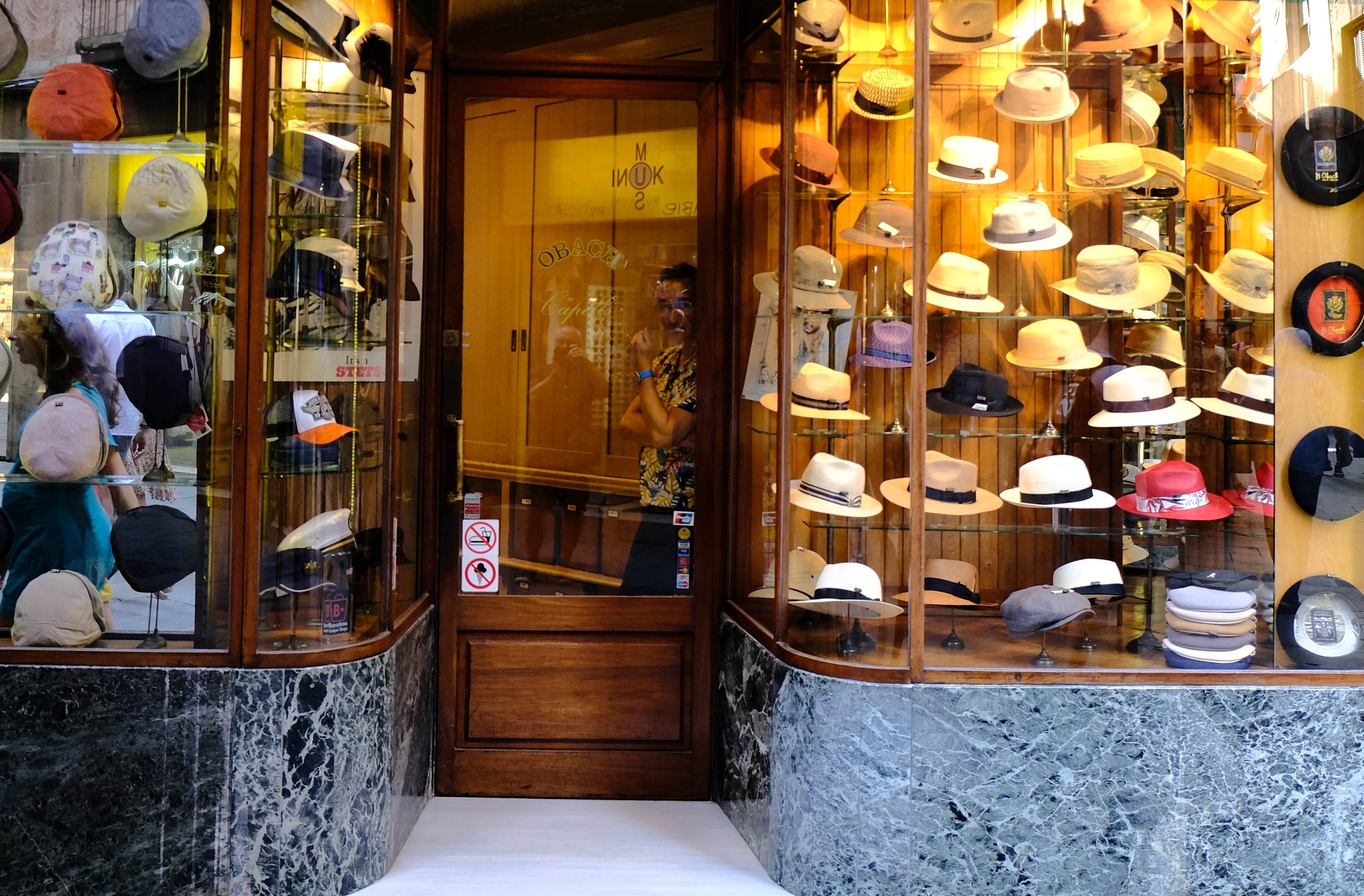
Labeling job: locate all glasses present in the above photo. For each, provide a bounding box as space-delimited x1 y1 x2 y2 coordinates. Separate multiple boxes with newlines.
654 300 694 313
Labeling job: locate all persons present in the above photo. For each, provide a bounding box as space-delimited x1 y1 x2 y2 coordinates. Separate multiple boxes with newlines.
512 325 610 582
1065 325 1353 639
620 260 697 591
0 258 170 631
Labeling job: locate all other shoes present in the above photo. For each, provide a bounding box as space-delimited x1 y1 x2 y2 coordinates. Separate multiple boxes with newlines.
1324 460 1345 478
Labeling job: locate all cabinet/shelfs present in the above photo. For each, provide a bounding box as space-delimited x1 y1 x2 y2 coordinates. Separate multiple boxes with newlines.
0 93 397 669
747 51 1274 686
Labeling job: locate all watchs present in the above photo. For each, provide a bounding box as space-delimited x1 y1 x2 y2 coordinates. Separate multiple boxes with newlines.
633 369 655 384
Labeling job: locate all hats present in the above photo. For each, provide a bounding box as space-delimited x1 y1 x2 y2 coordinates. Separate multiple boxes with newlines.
1281 106 1364 207
1064 324 1201 428
992 66 1080 124
1116 460 1234 520
760 362 871 420
844 67 915 121
906 0 1017 54
1290 261 1364 357
981 197 1073 251
1122 1 1275 568
848 319 936 368
838 199 914 247
752 245 852 310
748 547 1364 671
1046 244 1172 311
0 0 421 645
926 363 1024 418
772 451 1116 518
1065 141 1156 189
903 252 1006 313
763 0 847 49
1287 425 1364 522
926 135 1009 184
1069 0 1174 52
759 132 845 190
1006 317 1102 371
